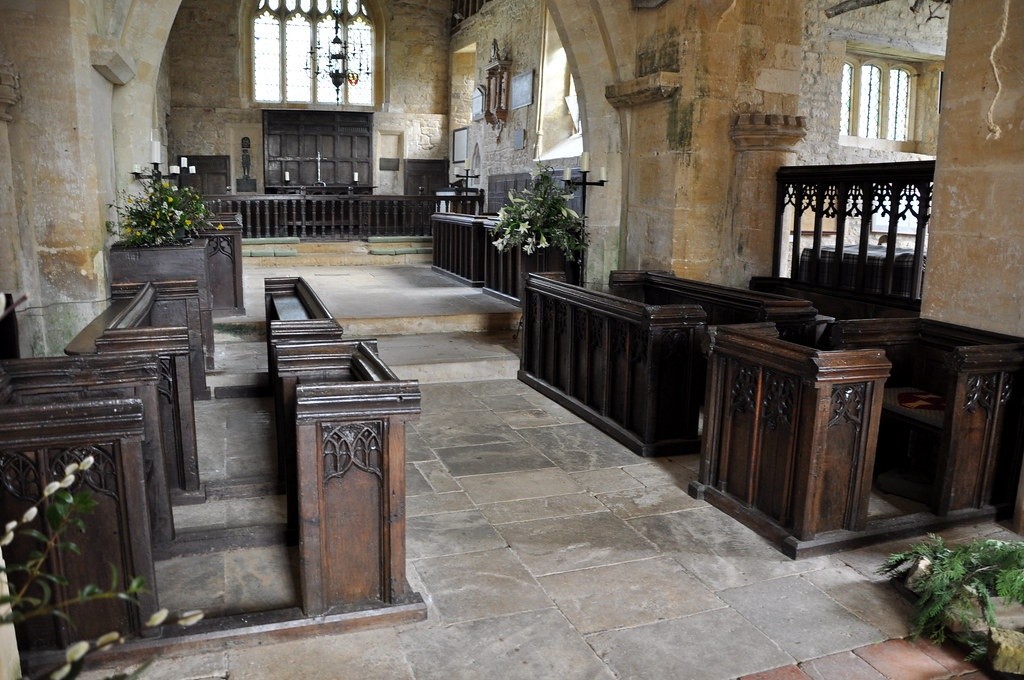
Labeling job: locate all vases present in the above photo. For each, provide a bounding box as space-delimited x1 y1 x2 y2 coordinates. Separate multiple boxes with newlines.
155 225 186 239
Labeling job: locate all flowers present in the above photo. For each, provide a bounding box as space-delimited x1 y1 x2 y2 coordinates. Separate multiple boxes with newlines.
490 156 590 263
106 169 225 247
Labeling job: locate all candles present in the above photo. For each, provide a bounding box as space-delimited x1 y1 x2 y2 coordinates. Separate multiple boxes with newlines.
284 171 290 180
354 172 358 181
170 166 180 174
180 157 188 168
152 140 161 162
132 164 142 173
189 166 196 174
599 166 608 181
581 152 590 171
562 166 572 180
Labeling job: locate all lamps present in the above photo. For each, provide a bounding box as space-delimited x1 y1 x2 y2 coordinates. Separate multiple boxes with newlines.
303 0 370 107
